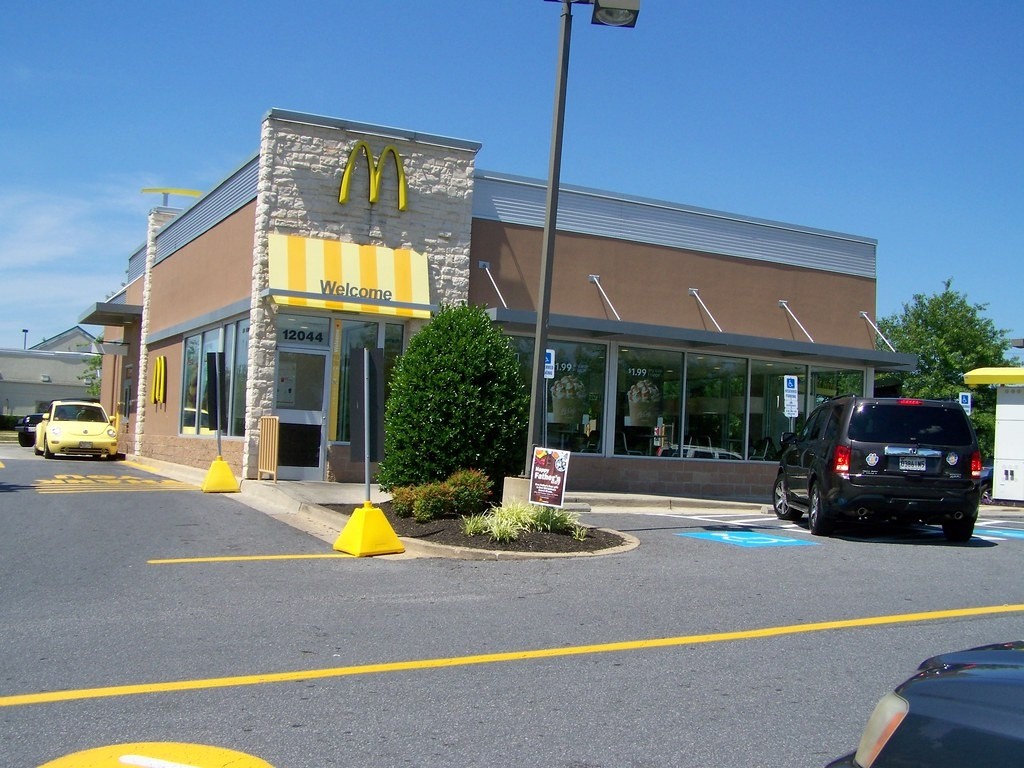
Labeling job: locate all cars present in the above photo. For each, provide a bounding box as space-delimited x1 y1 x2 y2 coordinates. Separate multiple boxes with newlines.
34 400 119 461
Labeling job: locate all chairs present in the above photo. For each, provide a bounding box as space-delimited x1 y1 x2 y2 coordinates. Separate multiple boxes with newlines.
77 410 90 421
557 427 775 461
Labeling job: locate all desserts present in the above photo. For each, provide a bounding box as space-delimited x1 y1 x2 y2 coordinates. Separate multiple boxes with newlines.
627 380 661 426
551 375 587 424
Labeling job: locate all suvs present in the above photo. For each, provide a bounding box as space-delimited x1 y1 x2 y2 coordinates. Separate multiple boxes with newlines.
657 444 742 460
14 398 100 447
772 394 982 543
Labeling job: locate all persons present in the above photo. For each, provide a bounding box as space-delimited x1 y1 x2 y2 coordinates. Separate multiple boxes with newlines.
56 409 68 420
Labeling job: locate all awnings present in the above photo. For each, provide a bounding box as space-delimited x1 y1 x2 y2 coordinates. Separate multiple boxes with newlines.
963 368 1024 384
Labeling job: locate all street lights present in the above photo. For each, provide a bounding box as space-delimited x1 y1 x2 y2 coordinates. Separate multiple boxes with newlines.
516 0 644 479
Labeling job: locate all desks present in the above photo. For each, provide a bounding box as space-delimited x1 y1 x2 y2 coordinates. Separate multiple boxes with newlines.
722 437 745 461
554 429 578 449
643 433 668 456
